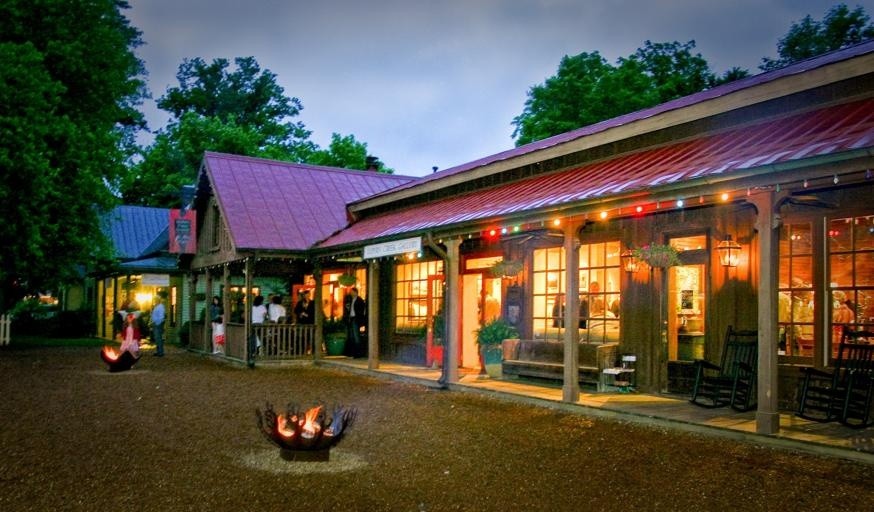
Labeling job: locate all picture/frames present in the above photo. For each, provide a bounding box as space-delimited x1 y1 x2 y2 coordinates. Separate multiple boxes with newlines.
678 289 698 316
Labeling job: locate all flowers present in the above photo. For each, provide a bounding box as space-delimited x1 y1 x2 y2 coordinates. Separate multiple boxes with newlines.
636 243 683 270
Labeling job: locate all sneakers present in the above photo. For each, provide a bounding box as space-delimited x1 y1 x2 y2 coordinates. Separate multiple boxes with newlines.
258 347 263 358
154 352 164 357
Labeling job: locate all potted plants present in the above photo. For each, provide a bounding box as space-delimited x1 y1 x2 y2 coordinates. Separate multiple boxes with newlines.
324 315 348 356
475 314 520 378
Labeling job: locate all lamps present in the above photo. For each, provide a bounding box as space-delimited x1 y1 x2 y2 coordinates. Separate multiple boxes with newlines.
620 250 640 272
713 235 741 268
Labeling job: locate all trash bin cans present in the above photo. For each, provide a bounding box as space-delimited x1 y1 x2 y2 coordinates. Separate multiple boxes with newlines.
327 334 346 353
618 353 636 386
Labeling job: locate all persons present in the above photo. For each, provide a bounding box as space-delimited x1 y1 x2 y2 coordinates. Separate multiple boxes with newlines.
206 288 314 357
477 288 501 328
145 294 168 358
342 287 365 358
775 275 865 359
118 313 141 355
550 280 620 332
114 298 141 358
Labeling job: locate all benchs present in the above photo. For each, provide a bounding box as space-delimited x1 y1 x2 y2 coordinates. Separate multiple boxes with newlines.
504 338 619 393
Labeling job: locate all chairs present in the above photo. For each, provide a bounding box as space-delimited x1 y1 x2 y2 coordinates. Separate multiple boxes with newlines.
689 325 758 413
794 322 874 429
